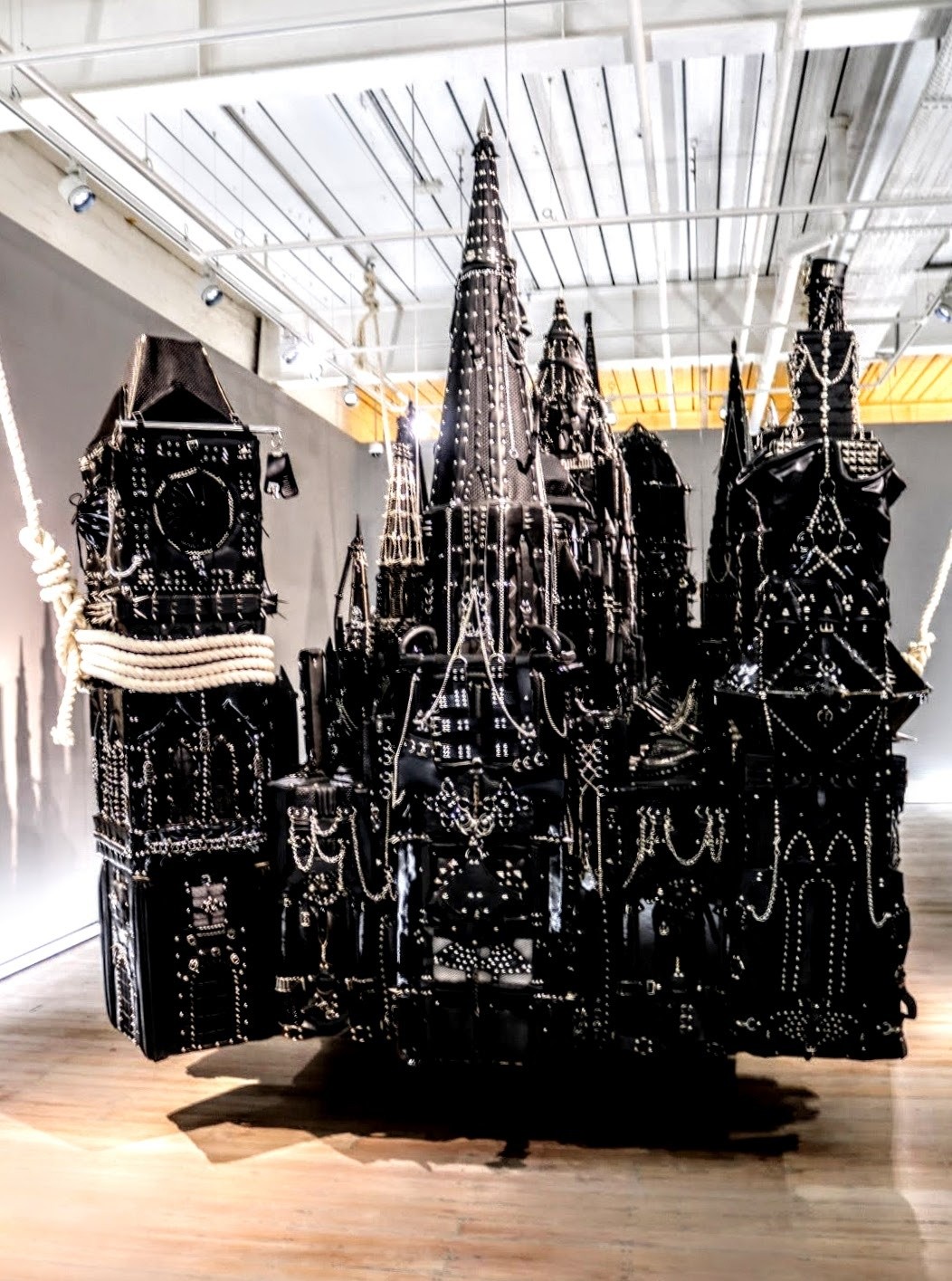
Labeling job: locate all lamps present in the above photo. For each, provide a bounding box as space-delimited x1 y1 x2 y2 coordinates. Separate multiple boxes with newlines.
341 376 358 407
196 264 224 305
56 159 96 211
282 332 301 365
